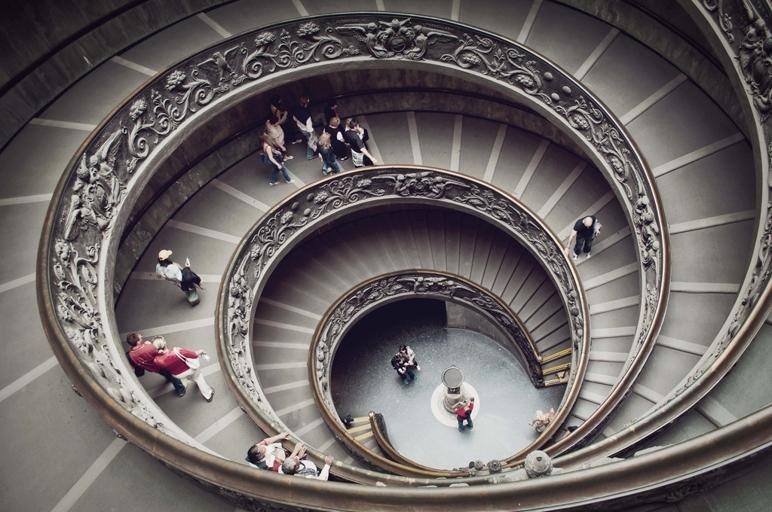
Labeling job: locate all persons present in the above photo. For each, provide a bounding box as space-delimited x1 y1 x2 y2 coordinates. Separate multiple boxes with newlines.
258 92 382 185
279 446 335 481
155 246 203 307
335 404 354 430
454 394 474 433
125 332 187 397
395 344 421 371
245 431 291 474
394 354 417 385
465 397 474 414
563 213 600 262
152 336 216 401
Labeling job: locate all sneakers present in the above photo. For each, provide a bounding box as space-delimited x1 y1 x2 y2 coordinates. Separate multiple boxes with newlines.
205 385 215 402
573 251 579 259
585 251 592 259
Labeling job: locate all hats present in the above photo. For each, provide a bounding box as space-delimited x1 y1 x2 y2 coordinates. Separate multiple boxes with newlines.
158 249 172 261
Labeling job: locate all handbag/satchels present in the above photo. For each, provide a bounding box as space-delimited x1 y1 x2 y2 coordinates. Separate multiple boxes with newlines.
173 346 199 371
258 144 285 169
124 352 145 378
